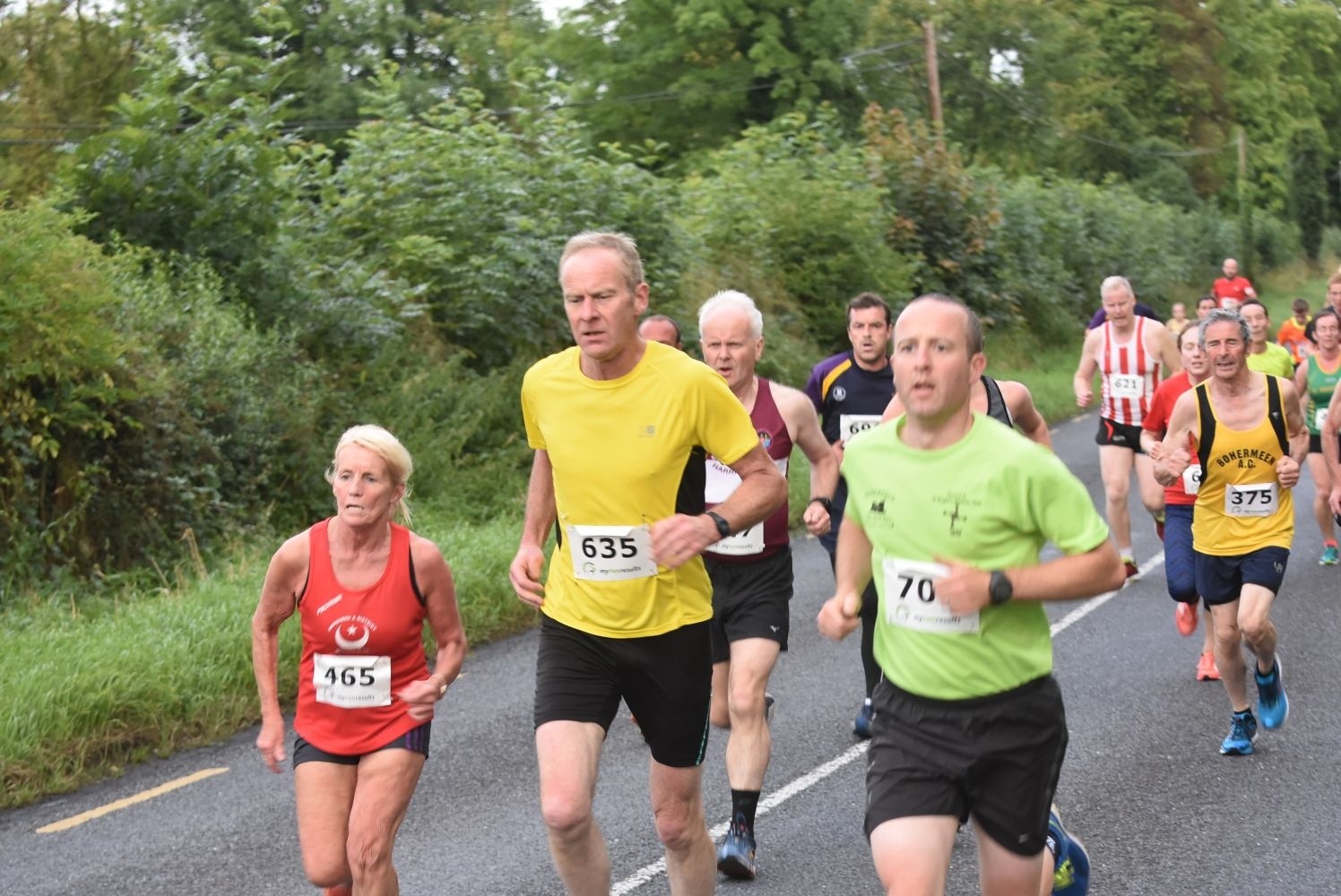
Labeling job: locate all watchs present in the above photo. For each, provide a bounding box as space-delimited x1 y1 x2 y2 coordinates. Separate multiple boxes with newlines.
806 496 833 517
986 570 1013 607
704 510 730 540
428 674 448 699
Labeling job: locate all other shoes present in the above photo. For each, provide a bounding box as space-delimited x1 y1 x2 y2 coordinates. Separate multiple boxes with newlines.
325 885 352 896
850 698 875 735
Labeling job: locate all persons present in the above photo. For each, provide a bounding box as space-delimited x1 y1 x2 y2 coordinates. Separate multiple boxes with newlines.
802 292 896 739
881 373 1054 453
815 293 1127 896
636 314 683 350
251 424 467 896
510 232 790 896
697 289 839 880
1075 257 1340 753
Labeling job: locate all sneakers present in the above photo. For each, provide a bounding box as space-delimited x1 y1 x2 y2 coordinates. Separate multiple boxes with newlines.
1318 545 1339 565
718 810 756 877
1196 652 1221 680
1176 601 1198 636
764 692 774 726
1219 712 1258 754
1254 652 1289 729
1047 805 1091 896
1155 515 1165 541
1123 559 1139 578
